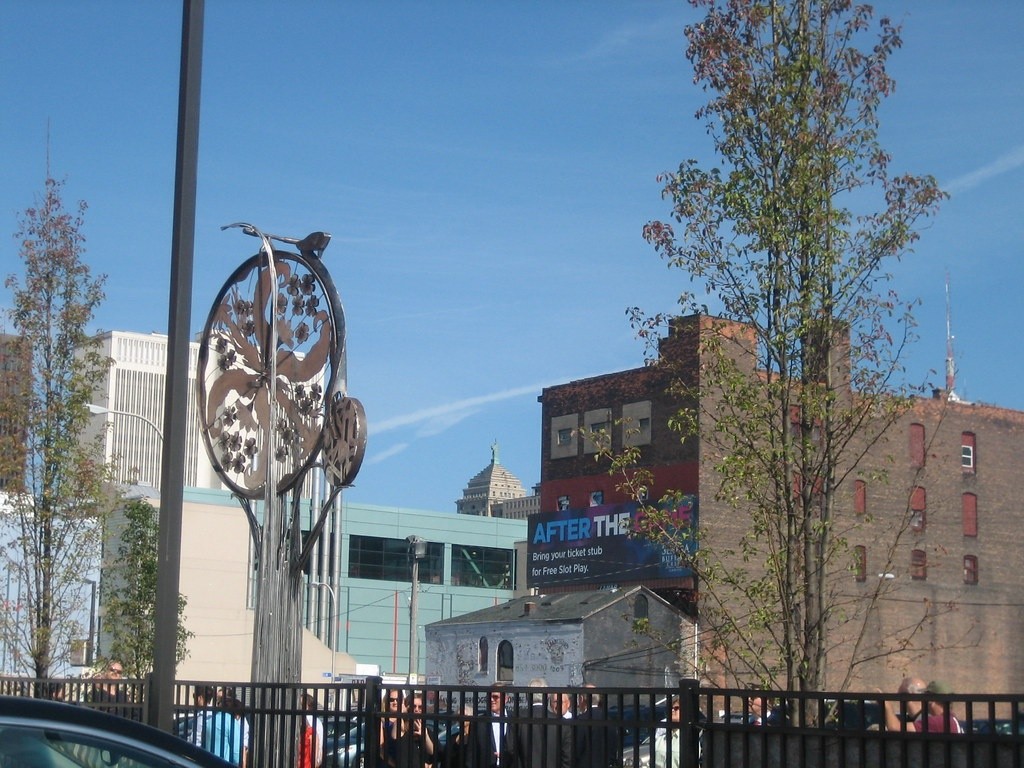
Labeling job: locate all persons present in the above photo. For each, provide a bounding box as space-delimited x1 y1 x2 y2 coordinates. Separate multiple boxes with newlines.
298 694 323 768
188 685 249 768
464 682 512 768
741 684 788 727
647 699 701 768
46 683 66 702
92 660 131 719
505 678 617 768
380 688 442 768
867 678 963 734
440 705 473 768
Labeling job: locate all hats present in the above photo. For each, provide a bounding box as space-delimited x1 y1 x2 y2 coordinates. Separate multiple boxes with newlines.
898 677 927 694
918 681 954 695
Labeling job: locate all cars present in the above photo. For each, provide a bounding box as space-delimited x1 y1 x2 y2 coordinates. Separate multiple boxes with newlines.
316 708 461 767
0 695 238 767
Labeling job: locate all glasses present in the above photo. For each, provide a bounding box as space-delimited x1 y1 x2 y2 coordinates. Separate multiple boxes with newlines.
672 706 679 711
414 705 422 709
109 667 124 675
491 694 500 701
389 698 398 702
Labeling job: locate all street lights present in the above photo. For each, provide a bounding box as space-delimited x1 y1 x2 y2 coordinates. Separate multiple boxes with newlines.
311 582 337 712
